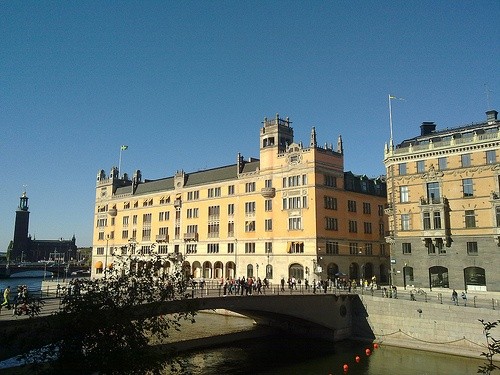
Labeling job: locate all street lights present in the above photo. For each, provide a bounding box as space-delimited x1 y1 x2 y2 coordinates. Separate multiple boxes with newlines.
105 234 111 268
234 238 239 278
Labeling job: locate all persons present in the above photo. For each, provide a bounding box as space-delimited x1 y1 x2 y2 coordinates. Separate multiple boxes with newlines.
392 285 398 298
193 276 378 294
1 286 11 308
461 292 466 306
452 289 457 305
